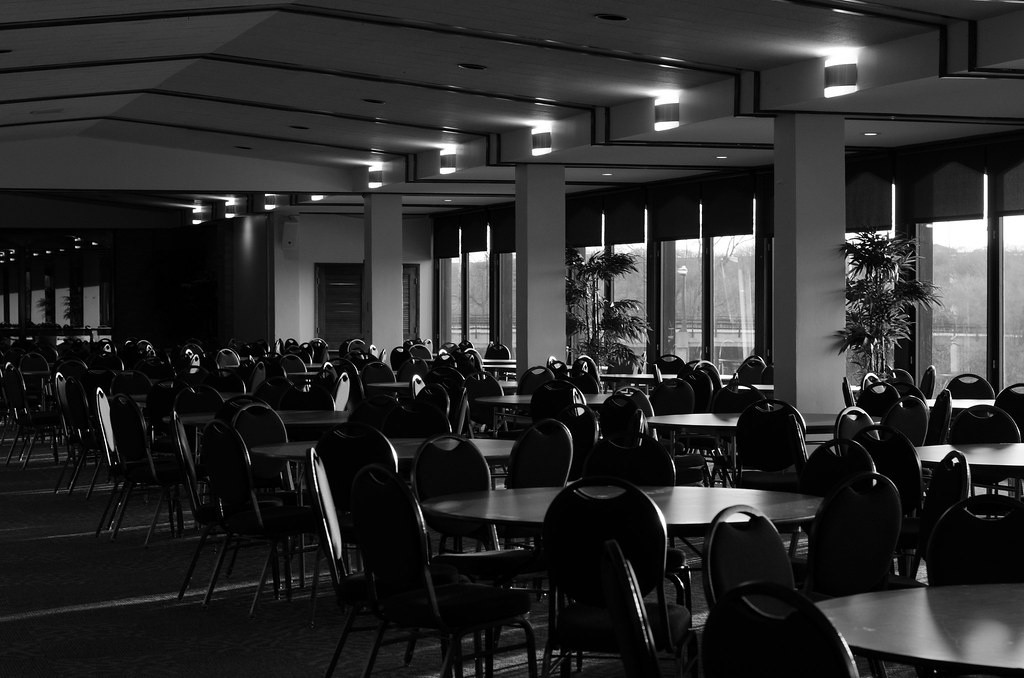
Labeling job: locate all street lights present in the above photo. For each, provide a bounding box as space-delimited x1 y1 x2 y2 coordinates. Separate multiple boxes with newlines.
676 265 688 331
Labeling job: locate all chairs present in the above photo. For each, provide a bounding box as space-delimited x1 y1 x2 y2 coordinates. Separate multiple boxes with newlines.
0 337 1024 678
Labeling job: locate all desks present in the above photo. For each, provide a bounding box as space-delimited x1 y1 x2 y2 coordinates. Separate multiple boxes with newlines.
814 582 1024 678
163 410 350 488
476 394 652 438
905 397 996 425
422 481 827 678
911 443 1024 520
371 378 521 390
598 372 739 392
249 437 517 573
646 412 880 488
483 358 517 379
